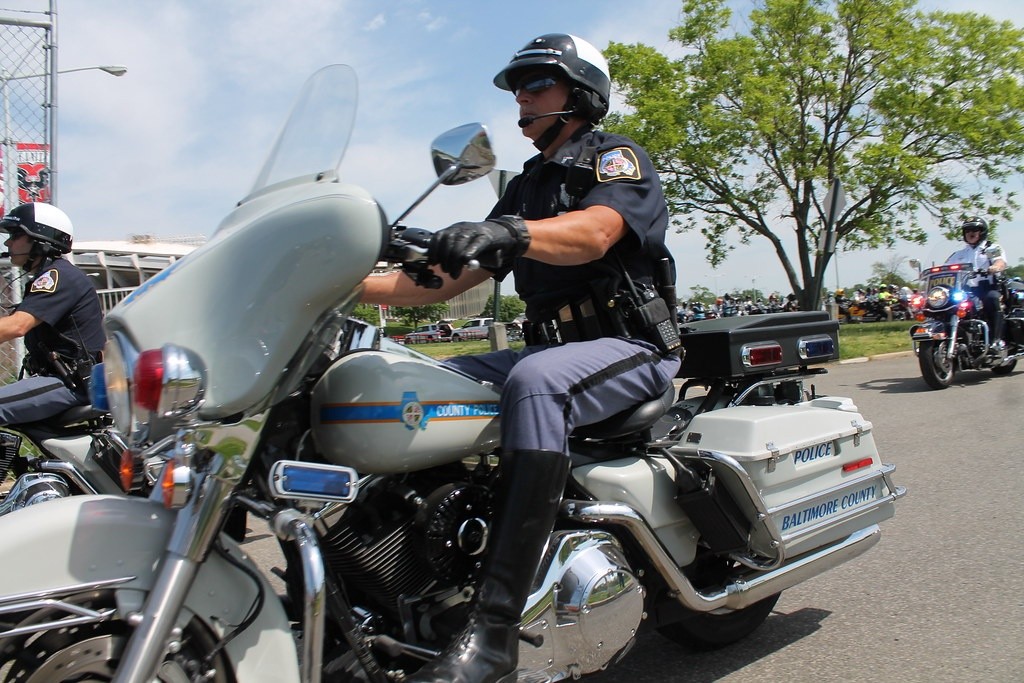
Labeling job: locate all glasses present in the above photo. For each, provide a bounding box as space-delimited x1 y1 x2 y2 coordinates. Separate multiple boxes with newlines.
8 230 25 240
514 67 562 98
964 227 981 233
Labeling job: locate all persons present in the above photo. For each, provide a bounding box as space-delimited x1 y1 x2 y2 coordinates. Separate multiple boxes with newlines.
0 202 107 427
677 290 800 322
835 283 912 322
356 30 681 683
943 216 1008 351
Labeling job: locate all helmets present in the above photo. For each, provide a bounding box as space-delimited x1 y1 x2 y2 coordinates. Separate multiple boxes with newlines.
493 33 611 118
960 216 989 243
0 202 74 253
680 281 899 310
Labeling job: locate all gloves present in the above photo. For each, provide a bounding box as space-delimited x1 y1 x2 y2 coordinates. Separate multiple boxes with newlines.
426 214 531 280
978 268 990 278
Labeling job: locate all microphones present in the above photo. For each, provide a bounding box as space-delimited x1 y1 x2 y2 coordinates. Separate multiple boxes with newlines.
971 235 980 238
518 110 573 128
2 252 43 258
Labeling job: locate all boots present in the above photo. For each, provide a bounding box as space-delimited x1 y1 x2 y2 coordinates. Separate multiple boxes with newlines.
402 450 570 683
989 311 1004 355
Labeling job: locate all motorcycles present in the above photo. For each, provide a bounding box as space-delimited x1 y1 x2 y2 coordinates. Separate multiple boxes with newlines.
1 402 142 516
1 61 910 683
675 289 908 324
910 245 1024 390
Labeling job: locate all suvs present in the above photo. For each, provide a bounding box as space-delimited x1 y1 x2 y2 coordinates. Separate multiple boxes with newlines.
403 313 528 342
